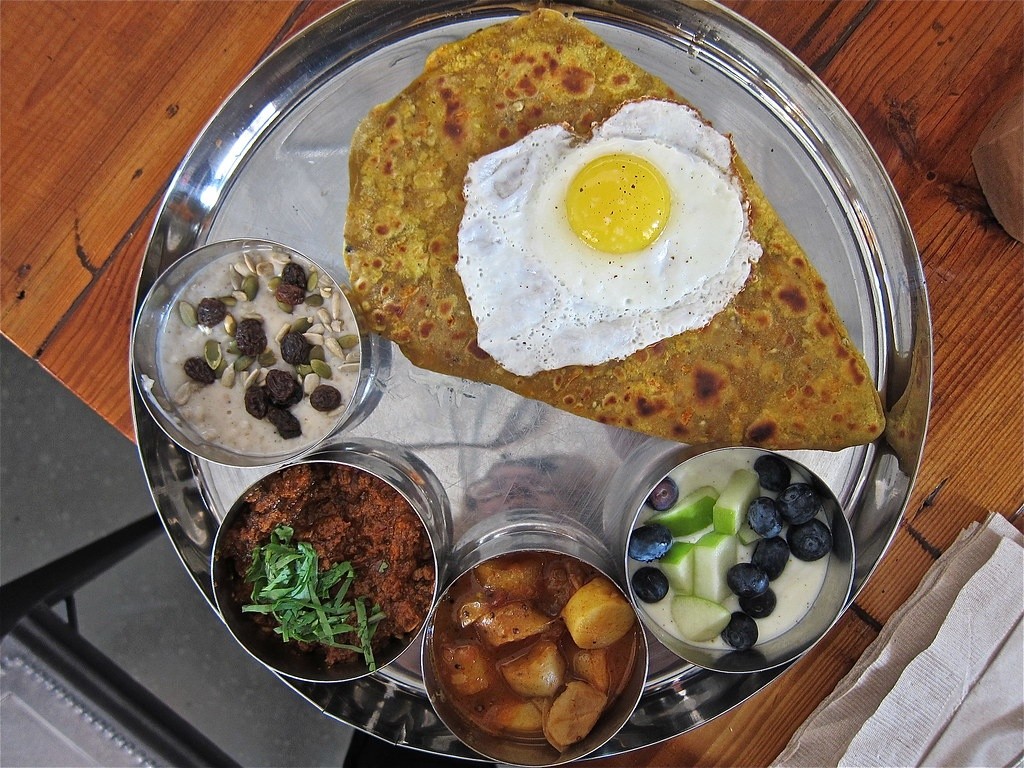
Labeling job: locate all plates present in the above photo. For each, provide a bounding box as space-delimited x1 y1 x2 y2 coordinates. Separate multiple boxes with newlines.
126 0 931 764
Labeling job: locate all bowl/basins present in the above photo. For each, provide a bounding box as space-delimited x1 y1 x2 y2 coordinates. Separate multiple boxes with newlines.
131 236 394 470
623 444 853 675
422 509 647 768
210 437 454 686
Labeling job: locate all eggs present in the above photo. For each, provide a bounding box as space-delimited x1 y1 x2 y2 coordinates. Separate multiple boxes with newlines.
452 95 765 379
